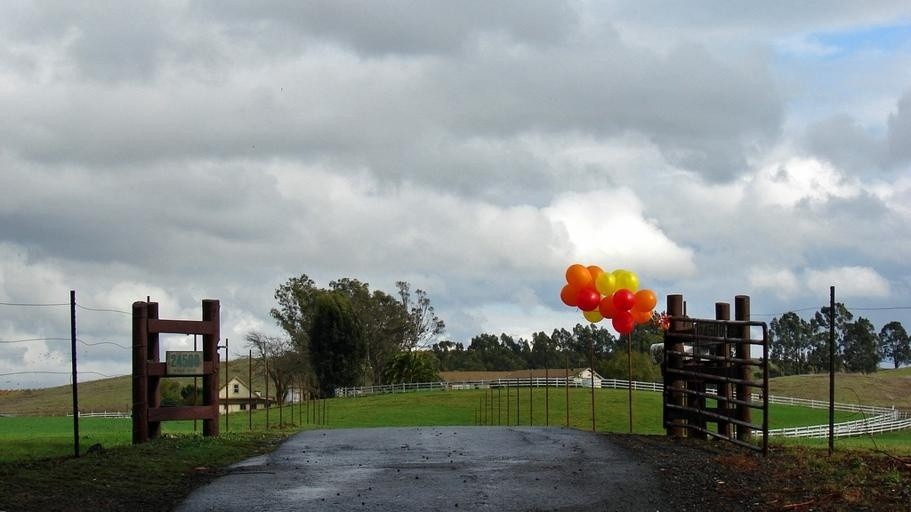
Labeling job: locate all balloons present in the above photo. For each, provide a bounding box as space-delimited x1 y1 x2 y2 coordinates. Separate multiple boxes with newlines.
561 263 659 335
